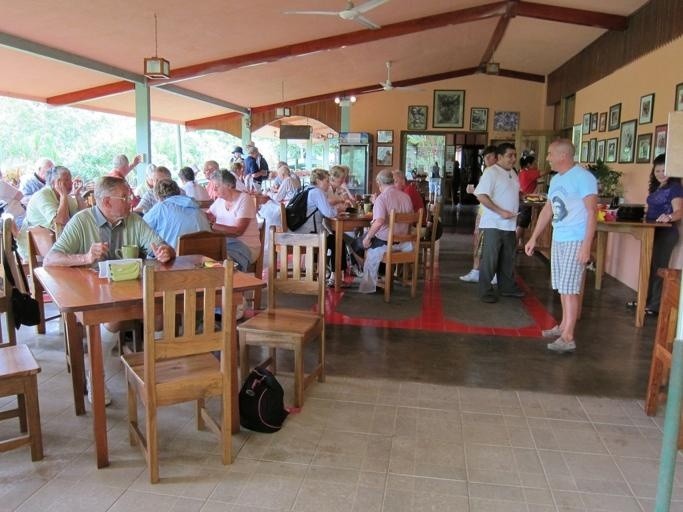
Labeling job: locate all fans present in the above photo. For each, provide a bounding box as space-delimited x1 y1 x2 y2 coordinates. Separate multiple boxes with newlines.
361 60 426 93
283 0 388 29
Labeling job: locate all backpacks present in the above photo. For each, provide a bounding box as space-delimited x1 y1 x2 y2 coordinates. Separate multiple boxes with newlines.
283 186 319 231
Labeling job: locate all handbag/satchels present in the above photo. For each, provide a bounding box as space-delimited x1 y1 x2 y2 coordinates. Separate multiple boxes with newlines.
3 232 41 330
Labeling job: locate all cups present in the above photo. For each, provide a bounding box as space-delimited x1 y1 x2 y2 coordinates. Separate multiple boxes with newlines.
363 204 370 213
114 244 138 259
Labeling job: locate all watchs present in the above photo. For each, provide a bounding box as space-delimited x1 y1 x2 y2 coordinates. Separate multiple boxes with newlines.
667 215 671 220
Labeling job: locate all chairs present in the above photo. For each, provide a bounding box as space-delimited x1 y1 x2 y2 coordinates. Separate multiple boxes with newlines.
364 207 423 303
120 260 233 483
236 226 325 408
0 213 16 344
238 218 266 311
395 202 440 281
0 343 44 463
26 231 61 334
174 230 227 261
280 202 319 255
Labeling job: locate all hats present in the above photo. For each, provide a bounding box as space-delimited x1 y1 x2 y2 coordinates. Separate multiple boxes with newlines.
245 141 255 148
232 146 244 155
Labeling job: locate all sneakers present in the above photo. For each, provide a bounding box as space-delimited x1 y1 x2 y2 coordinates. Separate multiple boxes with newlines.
542 325 561 337
480 295 497 303
500 289 528 298
235 297 248 321
86 374 112 407
327 278 351 289
459 269 480 283
491 273 498 285
546 337 576 351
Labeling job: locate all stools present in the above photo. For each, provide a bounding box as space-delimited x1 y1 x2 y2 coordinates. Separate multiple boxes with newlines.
645 268 683 451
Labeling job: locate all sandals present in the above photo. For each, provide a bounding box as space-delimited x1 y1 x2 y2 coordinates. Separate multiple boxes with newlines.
645 308 656 316
626 300 638 309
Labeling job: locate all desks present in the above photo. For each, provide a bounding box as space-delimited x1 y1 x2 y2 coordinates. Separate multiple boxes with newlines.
591 217 673 328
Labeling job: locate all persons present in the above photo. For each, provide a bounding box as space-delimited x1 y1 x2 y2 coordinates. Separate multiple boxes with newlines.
43 178 175 407
526 139 598 351
514 151 557 249
142 180 213 257
136 164 158 197
23 158 53 196
204 169 261 319
0 171 23 237
371 170 426 242
177 166 210 201
228 163 246 194
628 152 683 315
249 147 269 177
274 163 300 188
244 157 262 191
459 146 498 284
134 167 173 213
229 147 244 168
430 162 441 196
352 170 414 281
203 161 222 199
106 155 142 206
295 169 353 281
411 166 421 181
474 143 525 303
19 167 86 260
324 167 354 210
270 169 300 199
143 182 205 255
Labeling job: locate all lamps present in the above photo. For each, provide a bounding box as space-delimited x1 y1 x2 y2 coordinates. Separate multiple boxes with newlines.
277 81 290 118
144 16 170 79
582 156 625 205
486 47 499 75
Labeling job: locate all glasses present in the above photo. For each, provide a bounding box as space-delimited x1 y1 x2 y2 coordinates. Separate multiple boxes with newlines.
110 194 135 203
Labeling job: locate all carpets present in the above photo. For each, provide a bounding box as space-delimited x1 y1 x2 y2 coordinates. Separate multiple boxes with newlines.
313 232 563 338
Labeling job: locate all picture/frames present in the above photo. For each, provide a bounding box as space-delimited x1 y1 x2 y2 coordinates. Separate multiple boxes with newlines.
599 112 607 132
589 138 597 163
605 137 618 163
674 83 683 112
376 146 393 166
582 141 589 162
653 124 668 160
608 103 621 131
583 113 590 135
470 107 489 131
636 133 652 163
407 105 427 130
618 119 637 164
376 130 394 143
432 89 465 128
639 93 654 124
596 139 605 163
493 110 520 132
591 112 598 130
